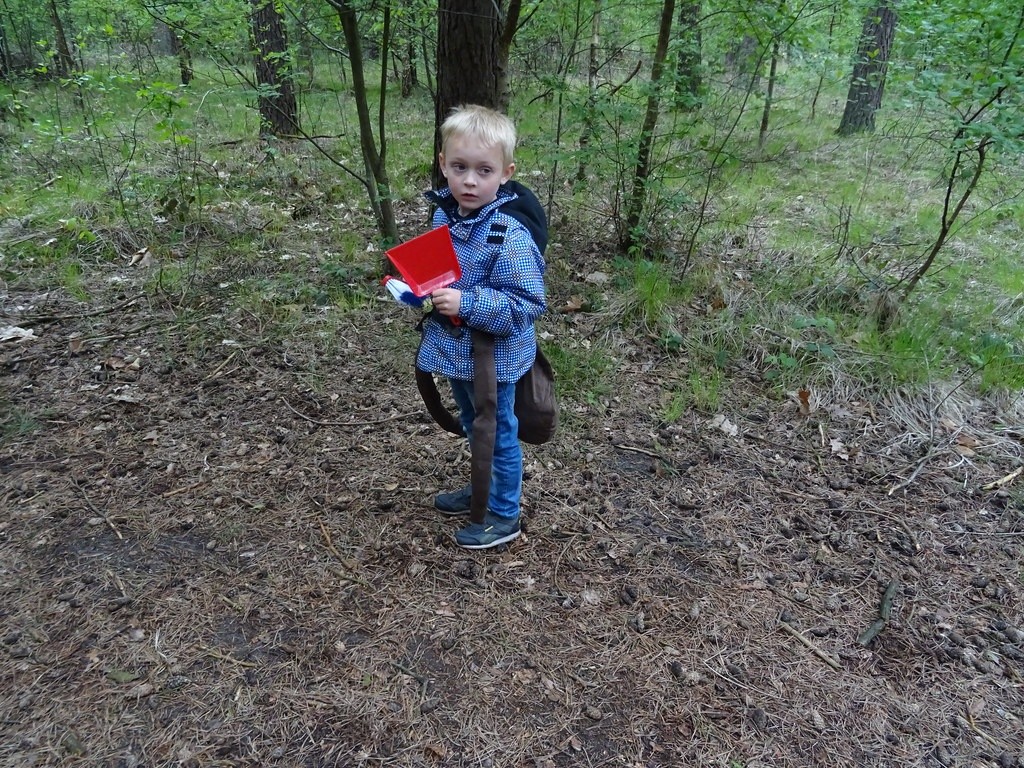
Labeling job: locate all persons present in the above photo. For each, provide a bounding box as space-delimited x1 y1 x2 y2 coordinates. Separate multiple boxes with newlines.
416 105 546 551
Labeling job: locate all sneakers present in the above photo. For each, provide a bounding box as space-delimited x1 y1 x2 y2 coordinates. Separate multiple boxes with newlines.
452 506 522 549
433 483 475 515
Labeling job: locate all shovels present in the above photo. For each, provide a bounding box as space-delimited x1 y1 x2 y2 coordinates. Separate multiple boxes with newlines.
385 224 468 329
